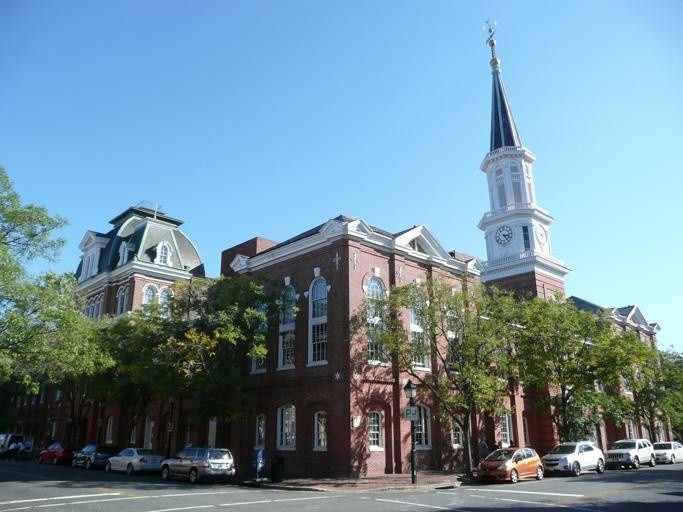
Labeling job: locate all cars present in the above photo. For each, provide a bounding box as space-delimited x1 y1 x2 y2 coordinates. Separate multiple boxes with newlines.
38 443 237 483
477 439 683 483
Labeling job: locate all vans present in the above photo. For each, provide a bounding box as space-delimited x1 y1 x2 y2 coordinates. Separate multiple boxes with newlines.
0 433 33 459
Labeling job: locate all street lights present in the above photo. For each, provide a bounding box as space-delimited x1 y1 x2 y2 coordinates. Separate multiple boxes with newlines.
165 396 177 457
404 379 417 484
